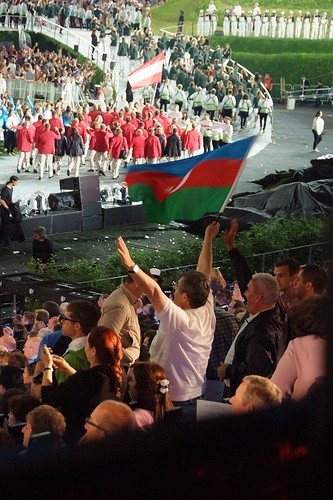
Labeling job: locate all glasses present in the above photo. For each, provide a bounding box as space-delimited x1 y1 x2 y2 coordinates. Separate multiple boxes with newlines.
58 315 77 325
84 417 105 432
299 265 311 275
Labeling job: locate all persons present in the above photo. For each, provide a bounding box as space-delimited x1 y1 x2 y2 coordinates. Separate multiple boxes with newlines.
0 219 333 456
0 175 20 247
0 0 333 180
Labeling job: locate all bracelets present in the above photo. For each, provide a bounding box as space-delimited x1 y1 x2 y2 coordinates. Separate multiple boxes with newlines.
43 368 54 371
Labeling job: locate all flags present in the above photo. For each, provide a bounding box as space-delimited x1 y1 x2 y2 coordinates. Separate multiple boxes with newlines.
126 50 165 89
124 136 257 225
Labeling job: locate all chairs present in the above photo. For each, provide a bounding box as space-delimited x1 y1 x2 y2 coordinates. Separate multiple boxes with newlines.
0 380 225 459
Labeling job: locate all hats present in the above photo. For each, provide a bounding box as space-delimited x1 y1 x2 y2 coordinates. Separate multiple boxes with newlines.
0 335 16 351
25 323 34 332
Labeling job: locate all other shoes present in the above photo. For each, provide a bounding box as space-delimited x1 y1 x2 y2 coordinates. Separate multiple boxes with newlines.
313 147 319 152
25 170 30 172
53 169 55 174
34 170 37 173
263 128 265 133
99 170 105 176
67 170 70 176
18 170 20 173
124 162 127 168
87 169 95 172
108 165 111 170
39 176 41 180
260 128 262 131
30 158 32 165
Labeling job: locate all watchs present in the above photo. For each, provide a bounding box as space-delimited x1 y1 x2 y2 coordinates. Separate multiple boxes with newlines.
127 264 139 273
